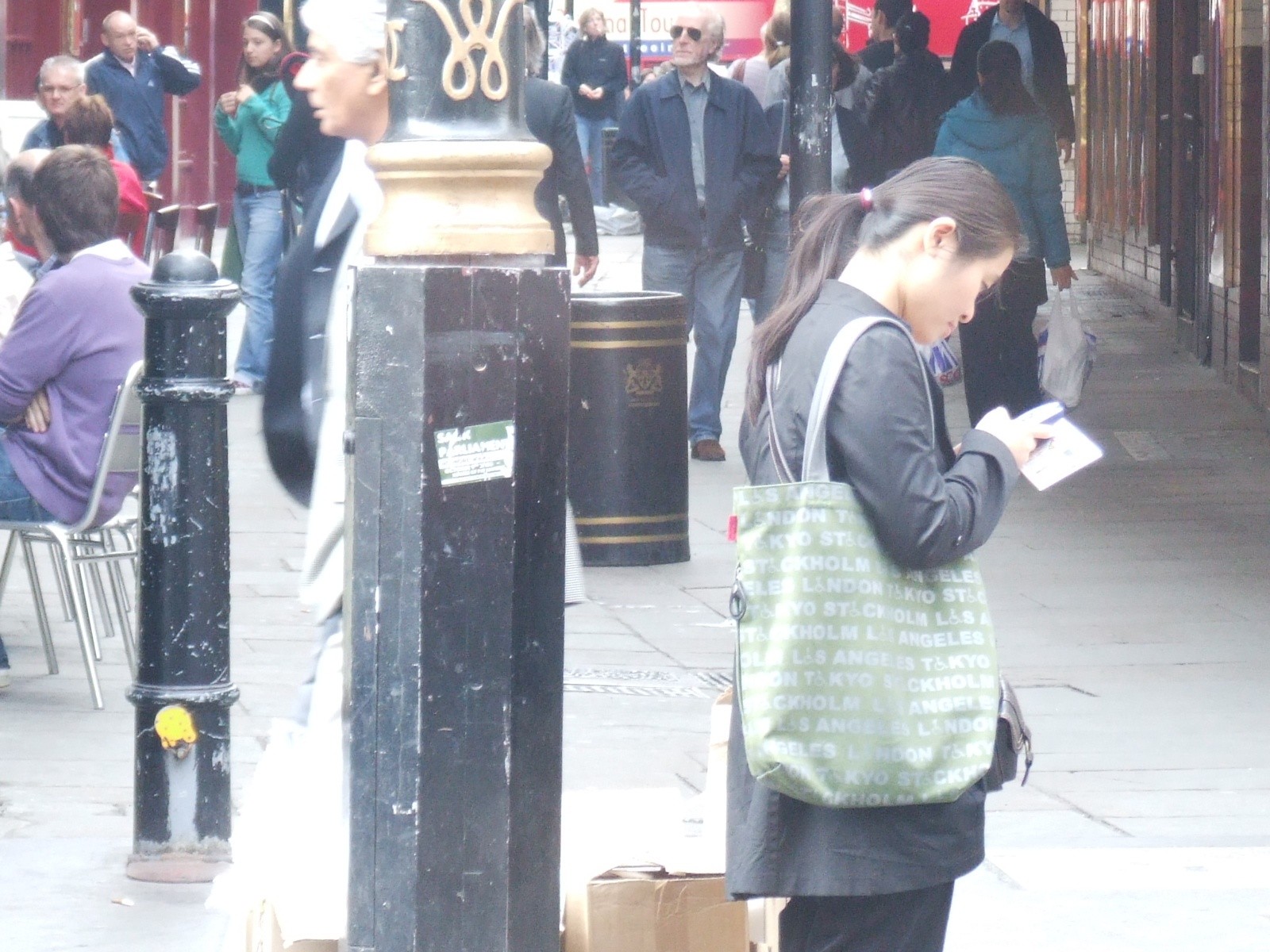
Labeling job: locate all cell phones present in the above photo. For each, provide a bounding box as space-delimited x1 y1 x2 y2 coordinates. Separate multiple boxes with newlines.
1011 400 1062 425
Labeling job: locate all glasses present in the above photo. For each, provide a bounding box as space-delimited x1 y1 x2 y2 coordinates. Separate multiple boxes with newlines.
669 23 713 42
40 83 83 92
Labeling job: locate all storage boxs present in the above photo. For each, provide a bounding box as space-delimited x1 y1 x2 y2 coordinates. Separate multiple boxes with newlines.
560 863 768 952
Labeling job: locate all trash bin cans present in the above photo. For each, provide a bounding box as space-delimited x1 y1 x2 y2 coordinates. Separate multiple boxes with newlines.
566 291 688 568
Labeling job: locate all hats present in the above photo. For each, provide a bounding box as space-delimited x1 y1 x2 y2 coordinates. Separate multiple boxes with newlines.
896 10 930 33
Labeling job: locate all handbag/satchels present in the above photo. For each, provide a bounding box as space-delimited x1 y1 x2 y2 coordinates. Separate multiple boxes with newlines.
984 685 1033 791
728 316 1001 810
1033 287 1098 409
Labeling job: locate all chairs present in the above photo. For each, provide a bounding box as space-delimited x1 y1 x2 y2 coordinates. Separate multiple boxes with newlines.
0 188 219 710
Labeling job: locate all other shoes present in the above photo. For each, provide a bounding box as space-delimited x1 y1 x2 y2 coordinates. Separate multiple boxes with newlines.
230 380 255 394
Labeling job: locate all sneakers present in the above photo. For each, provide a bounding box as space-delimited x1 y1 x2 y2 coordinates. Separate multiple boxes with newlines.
692 440 727 462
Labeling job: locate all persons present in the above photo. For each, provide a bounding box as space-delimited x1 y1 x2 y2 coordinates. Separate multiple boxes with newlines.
607 6 782 461
0 144 155 670
521 4 599 288
639 0 948 195
725 155 1058 952
950 0 1076 164
213 0 422 952
560 9 628 208
82 10 201 208
933 40 1079 431
744 37 880 328
0 54 149 354
